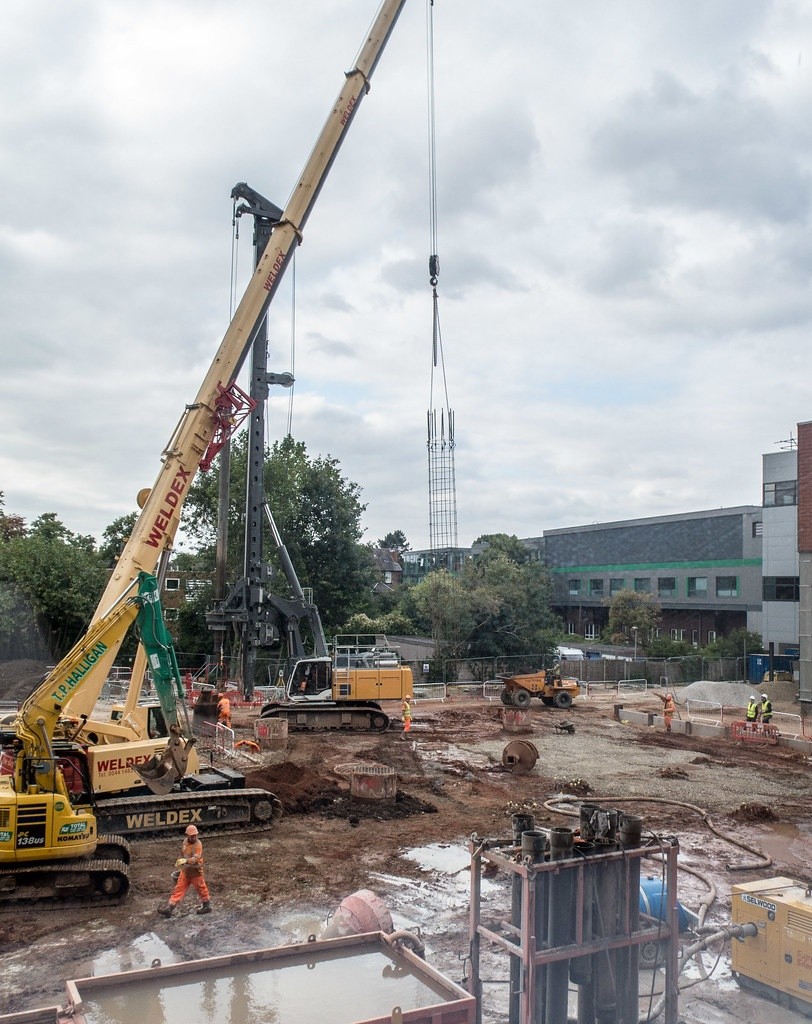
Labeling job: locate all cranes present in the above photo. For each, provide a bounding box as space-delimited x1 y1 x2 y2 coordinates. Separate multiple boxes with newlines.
180 182 333 709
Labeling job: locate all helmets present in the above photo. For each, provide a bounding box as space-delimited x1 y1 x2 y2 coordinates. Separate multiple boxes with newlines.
761 694 768 700
185 825 198 836
406 695 411 700
750 695 756 700
665 693 672 699
218 693 224 698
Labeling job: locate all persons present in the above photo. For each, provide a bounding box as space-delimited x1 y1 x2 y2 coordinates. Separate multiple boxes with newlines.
158 824 212 918
746 695 758 722
758 693 773 724
400 695 413 741
547 659 561 684
651 690 675 732
216 693 231 729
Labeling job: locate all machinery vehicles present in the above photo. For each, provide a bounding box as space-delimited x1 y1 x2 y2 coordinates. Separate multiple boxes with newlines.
2 574 197 911
2 1 412 913
257 646 412 734
495 658 581 709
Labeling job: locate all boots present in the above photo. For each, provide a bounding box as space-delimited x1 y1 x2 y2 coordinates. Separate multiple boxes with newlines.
196 902 212 915
157 904 175 918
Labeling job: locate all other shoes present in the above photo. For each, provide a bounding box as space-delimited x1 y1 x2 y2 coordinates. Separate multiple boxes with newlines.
406 735 412 740
400 736 406 741
